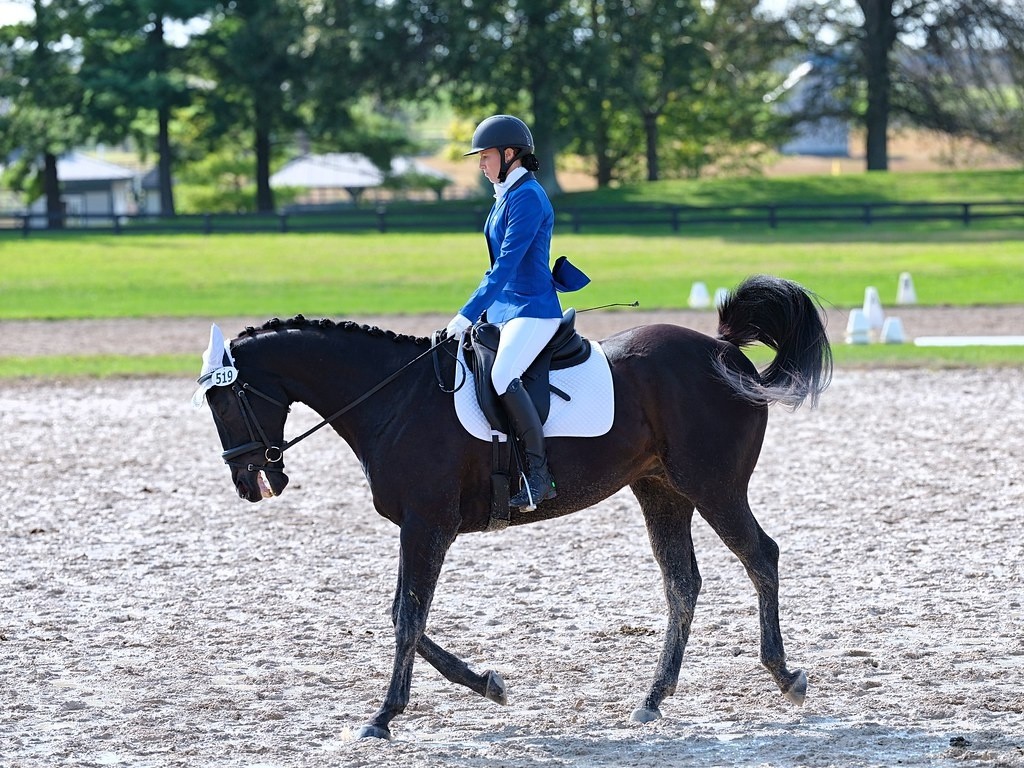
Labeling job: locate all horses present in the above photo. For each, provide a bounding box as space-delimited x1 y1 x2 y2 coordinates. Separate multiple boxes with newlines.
196 278 834 742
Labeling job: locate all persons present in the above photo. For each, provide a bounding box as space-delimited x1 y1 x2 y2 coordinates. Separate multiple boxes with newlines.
447 115 590 507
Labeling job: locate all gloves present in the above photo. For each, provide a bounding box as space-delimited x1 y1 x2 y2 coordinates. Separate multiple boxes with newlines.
445 313 472 343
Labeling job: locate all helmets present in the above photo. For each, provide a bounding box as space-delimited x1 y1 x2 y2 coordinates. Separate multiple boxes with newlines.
463 115 534 156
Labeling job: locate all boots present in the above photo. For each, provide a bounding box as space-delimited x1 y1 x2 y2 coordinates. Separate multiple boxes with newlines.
497 377 558 507
